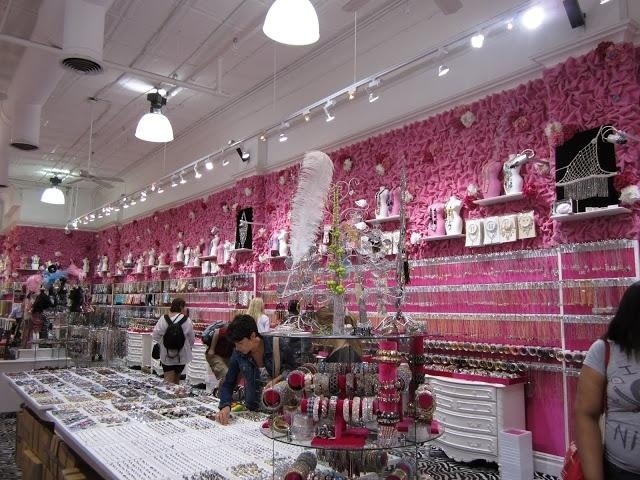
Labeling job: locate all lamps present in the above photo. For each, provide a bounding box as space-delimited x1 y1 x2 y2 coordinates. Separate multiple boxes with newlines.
40 175 67 205
133 90 175 143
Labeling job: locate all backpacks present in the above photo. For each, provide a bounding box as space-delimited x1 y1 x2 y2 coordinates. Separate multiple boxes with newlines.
163 314 188 351
201 318 232 343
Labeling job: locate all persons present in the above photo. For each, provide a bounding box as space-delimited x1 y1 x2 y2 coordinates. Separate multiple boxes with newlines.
68 281 84 324
152 298 195 385
205 298 298 426
28 286 58 330
573 282 640 480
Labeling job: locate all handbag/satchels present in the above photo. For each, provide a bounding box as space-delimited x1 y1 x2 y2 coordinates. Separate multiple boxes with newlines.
151 343 162 360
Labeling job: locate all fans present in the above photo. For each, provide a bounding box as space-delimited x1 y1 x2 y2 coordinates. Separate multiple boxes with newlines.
60 98 125 191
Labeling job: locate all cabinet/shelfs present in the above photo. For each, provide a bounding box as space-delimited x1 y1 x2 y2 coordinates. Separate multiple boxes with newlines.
186 344 219 392
260 326 445 480
126 331 152 369
423 374 527 464
